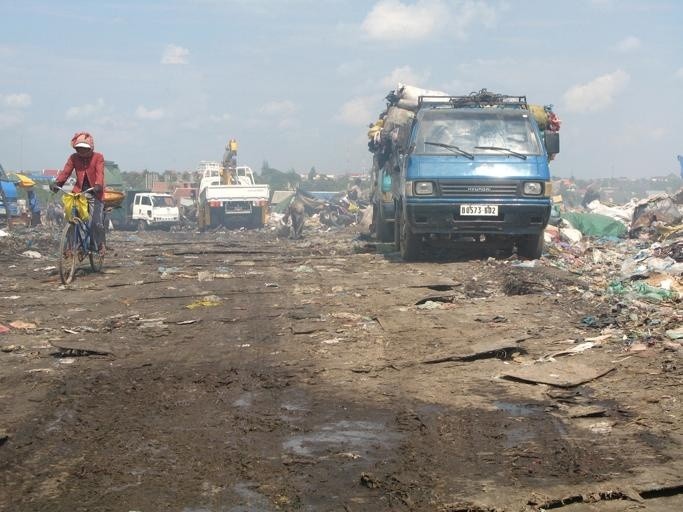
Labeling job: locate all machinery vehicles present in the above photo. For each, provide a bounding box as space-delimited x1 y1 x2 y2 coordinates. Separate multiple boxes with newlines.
193 138 276 230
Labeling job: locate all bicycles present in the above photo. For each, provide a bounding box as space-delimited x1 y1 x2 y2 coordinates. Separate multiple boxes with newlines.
50 191 107 283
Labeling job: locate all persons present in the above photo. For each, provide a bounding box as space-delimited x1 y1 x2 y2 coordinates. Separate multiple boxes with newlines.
50 132 106 257
348 179 364 198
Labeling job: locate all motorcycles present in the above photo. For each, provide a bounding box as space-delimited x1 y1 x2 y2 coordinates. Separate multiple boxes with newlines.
328 200 365 226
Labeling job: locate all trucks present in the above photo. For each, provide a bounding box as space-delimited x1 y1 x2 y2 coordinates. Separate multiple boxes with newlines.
0 162 18 216
371 93 552 261
103 175 179 230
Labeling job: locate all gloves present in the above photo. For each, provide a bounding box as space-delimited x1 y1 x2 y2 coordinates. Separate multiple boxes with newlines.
94 184 102 193
50 183 62 193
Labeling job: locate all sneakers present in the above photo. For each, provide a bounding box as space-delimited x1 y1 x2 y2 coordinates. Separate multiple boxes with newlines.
63 249 72 255
98 242 105 257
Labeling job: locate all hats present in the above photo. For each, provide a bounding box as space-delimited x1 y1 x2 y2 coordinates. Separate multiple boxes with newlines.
73 135 90 148
